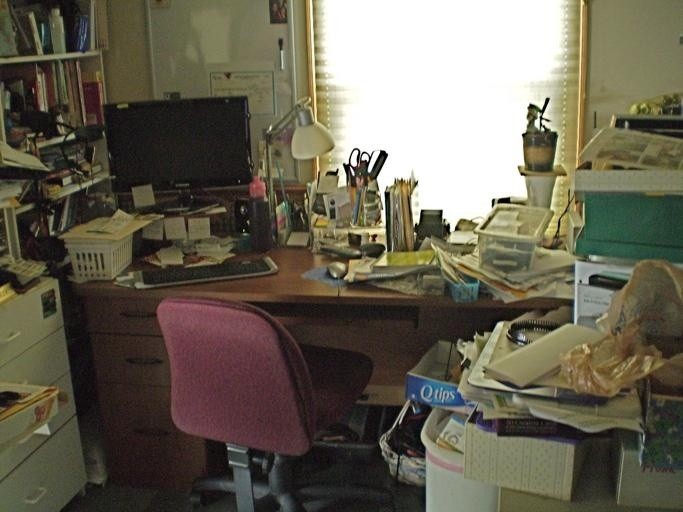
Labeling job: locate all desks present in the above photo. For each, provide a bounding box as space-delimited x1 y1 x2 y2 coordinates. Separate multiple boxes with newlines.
60 242 575 494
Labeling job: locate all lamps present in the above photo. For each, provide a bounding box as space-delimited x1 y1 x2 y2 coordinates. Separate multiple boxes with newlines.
261 96 336 246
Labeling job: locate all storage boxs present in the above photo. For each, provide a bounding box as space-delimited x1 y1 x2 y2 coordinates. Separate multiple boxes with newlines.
575 115 683 327
405 339 683 511
473 203 556 275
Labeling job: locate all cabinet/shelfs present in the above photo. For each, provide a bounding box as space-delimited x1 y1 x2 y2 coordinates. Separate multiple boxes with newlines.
0 276 89 512
0 0 117 276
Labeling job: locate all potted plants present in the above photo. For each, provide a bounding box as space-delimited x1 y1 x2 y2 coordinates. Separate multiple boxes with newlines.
523 97 558 172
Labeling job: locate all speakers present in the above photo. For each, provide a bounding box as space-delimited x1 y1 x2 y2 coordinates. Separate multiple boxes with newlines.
234 197 273 253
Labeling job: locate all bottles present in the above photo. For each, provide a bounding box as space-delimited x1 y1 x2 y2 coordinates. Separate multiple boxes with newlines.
247 176 274 254
49 8 66 54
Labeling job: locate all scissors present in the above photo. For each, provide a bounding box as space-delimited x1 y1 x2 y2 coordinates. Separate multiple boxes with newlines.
349 148 370 172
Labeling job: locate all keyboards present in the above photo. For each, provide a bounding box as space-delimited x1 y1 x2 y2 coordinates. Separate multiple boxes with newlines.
132 257 279 290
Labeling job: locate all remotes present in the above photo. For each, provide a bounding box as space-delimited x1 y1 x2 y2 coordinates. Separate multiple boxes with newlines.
320 245 362 259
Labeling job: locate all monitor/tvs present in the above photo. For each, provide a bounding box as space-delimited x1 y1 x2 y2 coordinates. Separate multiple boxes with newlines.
103 95 254 215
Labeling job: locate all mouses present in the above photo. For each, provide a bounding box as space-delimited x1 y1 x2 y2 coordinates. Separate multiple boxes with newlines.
326 261 347 279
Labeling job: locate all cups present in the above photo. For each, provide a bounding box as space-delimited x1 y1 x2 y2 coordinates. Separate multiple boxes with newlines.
67 14 89 50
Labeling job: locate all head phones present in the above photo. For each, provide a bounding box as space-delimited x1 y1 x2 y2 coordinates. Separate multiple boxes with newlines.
60 128 96 176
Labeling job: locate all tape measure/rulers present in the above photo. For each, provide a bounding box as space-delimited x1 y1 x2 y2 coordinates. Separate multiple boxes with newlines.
370 151 387 180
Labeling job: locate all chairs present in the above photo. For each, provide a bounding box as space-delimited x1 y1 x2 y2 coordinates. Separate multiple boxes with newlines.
155 295 373 512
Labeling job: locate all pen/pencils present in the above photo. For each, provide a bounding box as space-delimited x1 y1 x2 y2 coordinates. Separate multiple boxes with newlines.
385 178 418 252
343 164 371 190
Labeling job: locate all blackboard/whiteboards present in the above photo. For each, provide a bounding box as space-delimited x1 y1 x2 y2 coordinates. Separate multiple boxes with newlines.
147 0 301 183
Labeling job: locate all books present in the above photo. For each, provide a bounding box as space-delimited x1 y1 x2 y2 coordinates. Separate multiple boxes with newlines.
1 59 105 149
1 141 109 263
578 125 683 172
341 171 587 306
0 0 90 58
